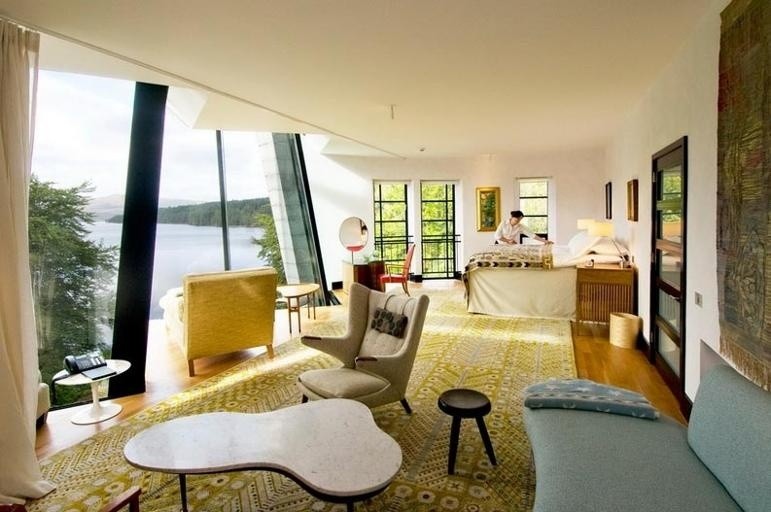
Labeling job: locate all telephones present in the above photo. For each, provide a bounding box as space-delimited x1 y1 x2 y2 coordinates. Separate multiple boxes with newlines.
64 352 107 374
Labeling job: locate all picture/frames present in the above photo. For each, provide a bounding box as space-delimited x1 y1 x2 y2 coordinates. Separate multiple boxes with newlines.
475 186 501 232
627 177 641 221
604 181 612 219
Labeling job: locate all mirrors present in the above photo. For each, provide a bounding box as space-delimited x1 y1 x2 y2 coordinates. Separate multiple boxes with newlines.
340 215 371 263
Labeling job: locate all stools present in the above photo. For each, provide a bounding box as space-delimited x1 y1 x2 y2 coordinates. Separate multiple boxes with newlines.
438 387 499 476
36 370 51 429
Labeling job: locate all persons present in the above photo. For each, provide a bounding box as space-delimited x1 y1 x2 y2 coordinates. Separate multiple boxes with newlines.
495 211 554 245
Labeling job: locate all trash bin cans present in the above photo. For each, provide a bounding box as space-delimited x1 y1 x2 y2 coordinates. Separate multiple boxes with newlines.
609 312 641 349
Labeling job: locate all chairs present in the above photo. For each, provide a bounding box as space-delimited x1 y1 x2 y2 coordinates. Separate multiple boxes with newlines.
298 282 432 417
381 243 419 296
1 479 143 512
160 264 279 378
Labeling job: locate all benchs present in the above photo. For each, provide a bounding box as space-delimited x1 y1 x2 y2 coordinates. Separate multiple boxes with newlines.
520 337 771 511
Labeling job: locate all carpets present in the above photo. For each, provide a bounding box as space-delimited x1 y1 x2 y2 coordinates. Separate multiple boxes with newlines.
20 288 582 512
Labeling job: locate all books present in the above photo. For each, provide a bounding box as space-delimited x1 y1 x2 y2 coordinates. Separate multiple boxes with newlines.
82 366 117 380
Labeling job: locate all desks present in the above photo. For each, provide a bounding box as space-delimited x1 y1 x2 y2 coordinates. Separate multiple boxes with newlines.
52 358 132 425
342 258 386 296
276 284 320 332
122 397 408 512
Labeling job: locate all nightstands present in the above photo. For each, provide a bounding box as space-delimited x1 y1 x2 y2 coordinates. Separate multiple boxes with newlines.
574 261 636 338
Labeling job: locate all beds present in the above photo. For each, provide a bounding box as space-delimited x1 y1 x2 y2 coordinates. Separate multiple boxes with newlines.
465 230 637 324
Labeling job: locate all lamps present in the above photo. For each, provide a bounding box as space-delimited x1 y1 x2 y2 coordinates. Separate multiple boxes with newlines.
576 217 595 230
662 220 683 238
590 219 628 264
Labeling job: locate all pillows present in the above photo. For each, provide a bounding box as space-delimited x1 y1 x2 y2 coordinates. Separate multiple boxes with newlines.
568 232 629 260
